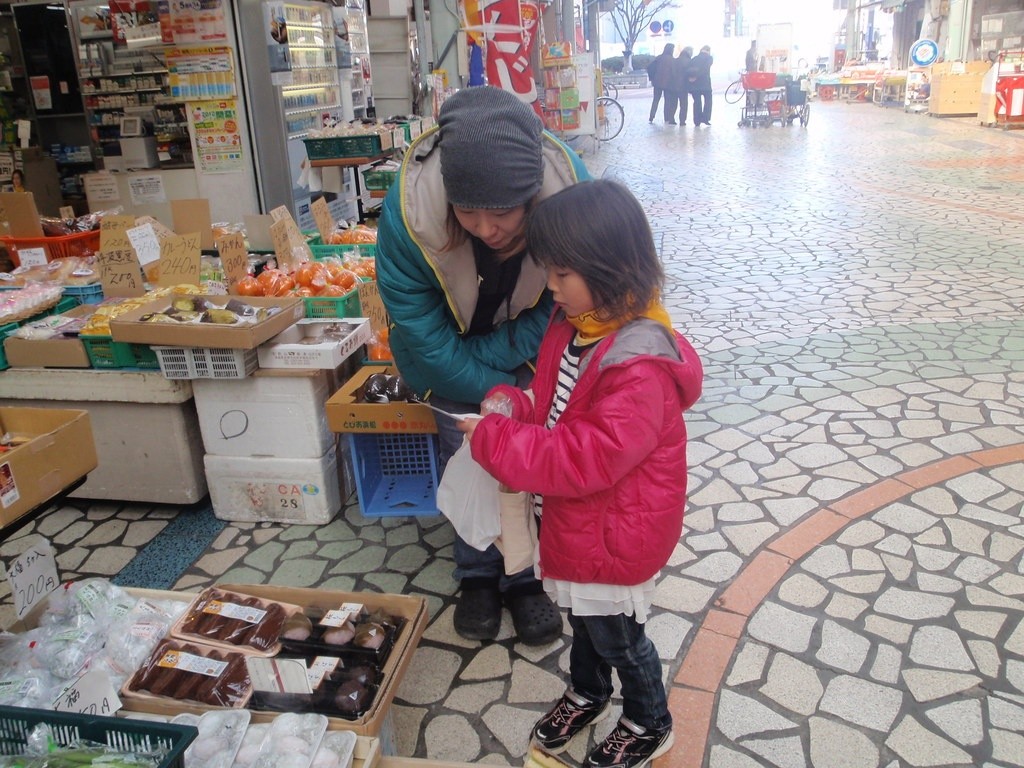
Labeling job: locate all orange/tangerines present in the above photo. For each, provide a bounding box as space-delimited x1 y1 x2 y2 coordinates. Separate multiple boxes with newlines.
325 225 378 244
369 326 394 362
237 257 376 299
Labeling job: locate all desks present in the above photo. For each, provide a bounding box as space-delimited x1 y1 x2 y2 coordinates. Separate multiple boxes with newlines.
811 78 875 103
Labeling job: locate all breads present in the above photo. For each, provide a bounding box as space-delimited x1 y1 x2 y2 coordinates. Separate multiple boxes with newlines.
0 255 99 289
80 282 209 334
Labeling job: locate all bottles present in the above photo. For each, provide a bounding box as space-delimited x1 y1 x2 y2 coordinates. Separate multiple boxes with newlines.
78 40 175 156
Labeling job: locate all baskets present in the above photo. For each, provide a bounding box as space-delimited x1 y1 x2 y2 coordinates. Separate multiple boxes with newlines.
347 432 441 518
0 225 388 383
301 114 435 191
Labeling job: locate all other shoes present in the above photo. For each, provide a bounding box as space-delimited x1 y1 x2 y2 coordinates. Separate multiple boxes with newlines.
670 120 677 125
680 120 687 126
695 122 701 127
649 117 654 122
665 120 670 125
702 120 711 125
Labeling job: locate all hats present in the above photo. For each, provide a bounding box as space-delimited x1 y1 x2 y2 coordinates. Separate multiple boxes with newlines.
438 85 543 209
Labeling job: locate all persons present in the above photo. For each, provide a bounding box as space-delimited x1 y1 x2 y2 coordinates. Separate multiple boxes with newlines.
456 180 704 768
745 40 758 106
374 87 596 646
646 43 713 127
760 56 765 71
914 74 930 99
7 169 24 191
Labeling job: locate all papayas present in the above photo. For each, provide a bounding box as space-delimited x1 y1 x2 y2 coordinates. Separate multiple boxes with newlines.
142 297 274 326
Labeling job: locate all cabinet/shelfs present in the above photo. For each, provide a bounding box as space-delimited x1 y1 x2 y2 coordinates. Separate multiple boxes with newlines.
314 140 414 224
333 0 375 122
64 0 192 167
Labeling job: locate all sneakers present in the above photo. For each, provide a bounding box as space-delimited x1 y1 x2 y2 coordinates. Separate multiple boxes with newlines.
583 713 674 768
529 686 613 755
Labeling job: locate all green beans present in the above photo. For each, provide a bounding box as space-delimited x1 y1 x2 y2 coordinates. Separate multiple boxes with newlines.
4 748 161 768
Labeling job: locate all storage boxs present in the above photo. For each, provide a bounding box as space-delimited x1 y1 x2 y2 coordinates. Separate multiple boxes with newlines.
302 117 436 190
0 216 442 533
0 575 430 768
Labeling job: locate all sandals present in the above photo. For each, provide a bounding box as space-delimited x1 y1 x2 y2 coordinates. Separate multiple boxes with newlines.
453 576 502 640
504 581 564 646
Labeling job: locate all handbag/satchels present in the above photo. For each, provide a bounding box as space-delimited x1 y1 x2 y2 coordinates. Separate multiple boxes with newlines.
435 401 502 552
494 483 538 577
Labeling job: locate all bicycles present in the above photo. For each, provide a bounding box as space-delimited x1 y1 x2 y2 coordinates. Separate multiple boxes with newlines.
724 69 746 103
563 82 625 142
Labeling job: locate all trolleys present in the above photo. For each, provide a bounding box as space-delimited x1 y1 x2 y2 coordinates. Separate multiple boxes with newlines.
783 76 811 127
